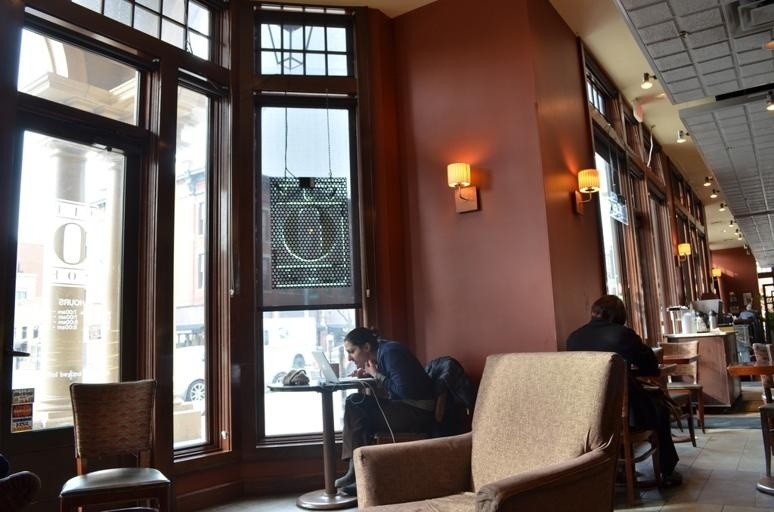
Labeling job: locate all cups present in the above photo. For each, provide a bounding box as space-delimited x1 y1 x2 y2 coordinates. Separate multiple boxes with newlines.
319 363 340 382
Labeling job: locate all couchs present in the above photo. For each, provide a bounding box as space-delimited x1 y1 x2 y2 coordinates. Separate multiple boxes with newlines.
353 351 626 512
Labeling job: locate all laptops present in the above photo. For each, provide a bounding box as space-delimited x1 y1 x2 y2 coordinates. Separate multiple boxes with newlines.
310 351 375 384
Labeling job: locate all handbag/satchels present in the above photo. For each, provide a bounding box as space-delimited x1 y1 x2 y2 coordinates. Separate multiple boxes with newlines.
283 369 310 385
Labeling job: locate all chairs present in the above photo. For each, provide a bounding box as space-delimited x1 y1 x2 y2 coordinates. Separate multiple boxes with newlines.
58 378 172 511
758 401 774 476
617 370 665 504
374 354 453 443
666 392 697 449
658 341 708 435
753 342 774 402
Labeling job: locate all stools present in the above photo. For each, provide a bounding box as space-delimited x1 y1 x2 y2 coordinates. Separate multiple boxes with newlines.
735 317 758 344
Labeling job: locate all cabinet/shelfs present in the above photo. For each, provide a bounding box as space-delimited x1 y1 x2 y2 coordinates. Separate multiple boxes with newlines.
666 331 742 410
718 324 754 381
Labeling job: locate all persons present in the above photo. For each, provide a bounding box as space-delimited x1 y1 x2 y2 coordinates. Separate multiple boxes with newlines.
565 295 683 490
335 327 435 495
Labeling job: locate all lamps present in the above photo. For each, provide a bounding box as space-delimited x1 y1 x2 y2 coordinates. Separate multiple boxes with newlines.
574 169 600 217
764 91 774 111
710 189 719 199
711 268 721 283
744 243 748 249
677 130 687 145
641 73 655 91
703 176 712 187
447 163 479 214
730 220 734 228
739 233 743 239
719 202 725 213
675 243 691 268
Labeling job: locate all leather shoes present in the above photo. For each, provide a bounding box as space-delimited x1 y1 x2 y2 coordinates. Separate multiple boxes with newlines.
616 469 642 484
336 458 356 487
342 483 357 498
658 473 682 488
